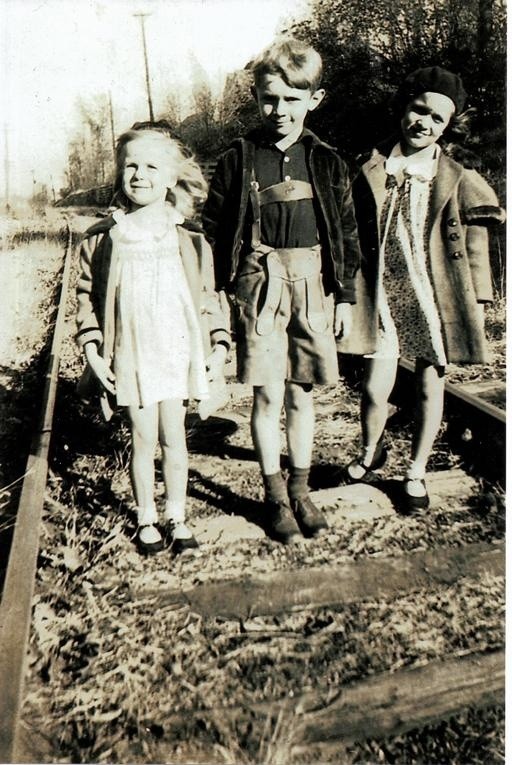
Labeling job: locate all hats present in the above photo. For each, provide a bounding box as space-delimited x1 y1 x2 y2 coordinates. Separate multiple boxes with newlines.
391 67 466 116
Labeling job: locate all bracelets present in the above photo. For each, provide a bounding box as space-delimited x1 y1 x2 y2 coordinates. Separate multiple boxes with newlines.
216 340 230 353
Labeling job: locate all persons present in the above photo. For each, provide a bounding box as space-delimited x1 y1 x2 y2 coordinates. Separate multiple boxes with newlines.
343 65 504 507
76 119 230 557
202 36 364 551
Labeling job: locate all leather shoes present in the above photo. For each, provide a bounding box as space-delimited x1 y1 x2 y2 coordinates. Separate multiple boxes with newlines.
402 471 430 512
166 520 198 548
344 449 387 484
134 523 163 555
293 493 330 536
266 500 303 545
